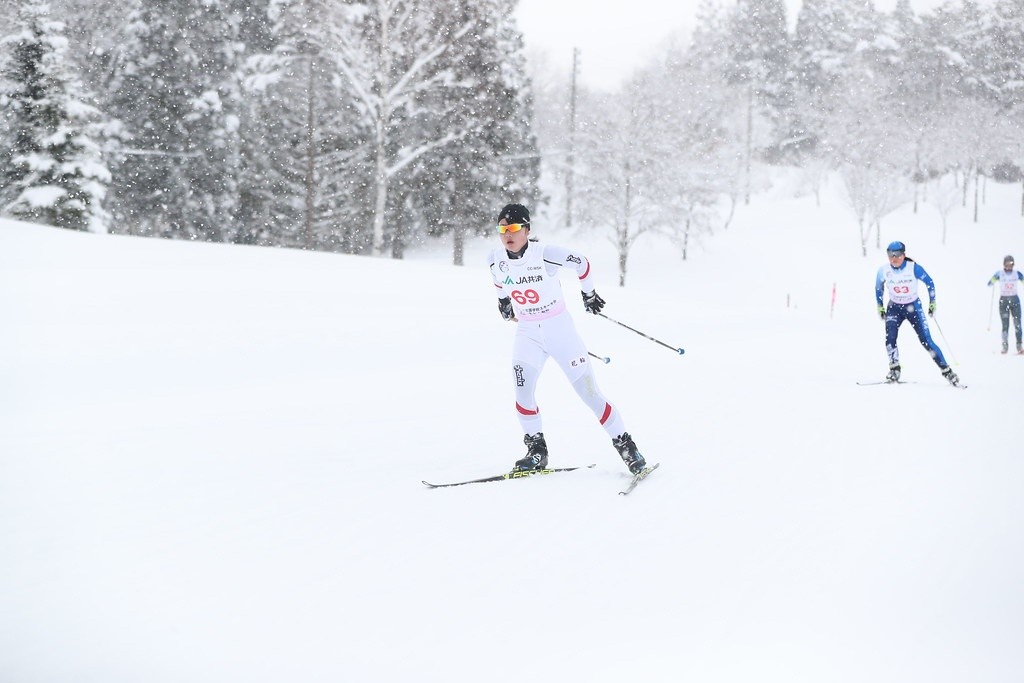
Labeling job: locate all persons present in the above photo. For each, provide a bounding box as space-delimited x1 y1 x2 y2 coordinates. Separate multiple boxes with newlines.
876 240 960 388
486 202 647 477
987 254 1024 354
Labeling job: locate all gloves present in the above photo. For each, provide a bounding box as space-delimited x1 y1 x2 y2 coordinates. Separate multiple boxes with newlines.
498 297 514 320
581 290 606 314
879 307 886 319
929 302 936 314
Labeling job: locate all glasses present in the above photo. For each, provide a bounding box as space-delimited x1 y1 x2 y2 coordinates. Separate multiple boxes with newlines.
887 249 904 258
496 223 528 234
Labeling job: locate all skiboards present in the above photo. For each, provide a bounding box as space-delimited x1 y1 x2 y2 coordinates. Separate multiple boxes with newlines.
421 463 660 496
855 380 968 389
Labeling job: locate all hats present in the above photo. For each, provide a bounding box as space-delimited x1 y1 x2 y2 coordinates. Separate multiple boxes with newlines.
498 204 531 233
887 241 906 253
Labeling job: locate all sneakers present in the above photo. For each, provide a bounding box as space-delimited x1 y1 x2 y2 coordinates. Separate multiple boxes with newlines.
1001 344 1008 353
886 366 900 381
1017 344 1023 352
613 432 646 472
515 432 548 468
943 368 959 384
